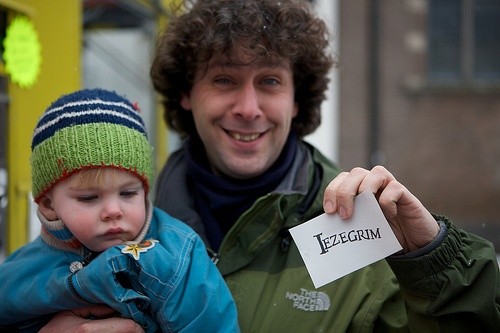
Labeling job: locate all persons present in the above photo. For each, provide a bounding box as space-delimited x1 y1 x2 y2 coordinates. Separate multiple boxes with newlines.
37 0 500 333
0 89 241 333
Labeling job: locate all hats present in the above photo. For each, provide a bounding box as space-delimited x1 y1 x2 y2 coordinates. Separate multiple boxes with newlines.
31 87 153 203
